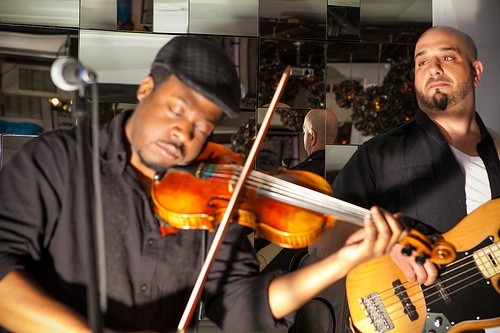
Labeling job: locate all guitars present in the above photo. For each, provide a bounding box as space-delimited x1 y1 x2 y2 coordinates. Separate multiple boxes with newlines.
345 197 500 333
229 118 258 154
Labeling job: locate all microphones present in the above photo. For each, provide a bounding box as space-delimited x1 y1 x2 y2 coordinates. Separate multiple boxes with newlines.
50 57 96 92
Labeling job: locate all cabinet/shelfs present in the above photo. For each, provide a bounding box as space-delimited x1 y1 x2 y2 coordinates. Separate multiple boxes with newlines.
0 0 260 85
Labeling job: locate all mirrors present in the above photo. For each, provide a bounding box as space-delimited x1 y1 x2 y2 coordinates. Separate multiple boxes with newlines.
259 0 432 186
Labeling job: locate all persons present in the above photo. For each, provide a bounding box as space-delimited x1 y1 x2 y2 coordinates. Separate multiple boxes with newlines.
287 108 339 178
309 26 500 287
0 36 410 333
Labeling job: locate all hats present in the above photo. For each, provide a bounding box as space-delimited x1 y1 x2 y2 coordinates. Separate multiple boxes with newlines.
151 34 242 118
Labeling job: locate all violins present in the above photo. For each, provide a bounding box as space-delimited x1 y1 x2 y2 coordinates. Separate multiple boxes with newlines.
151 141 458 267
